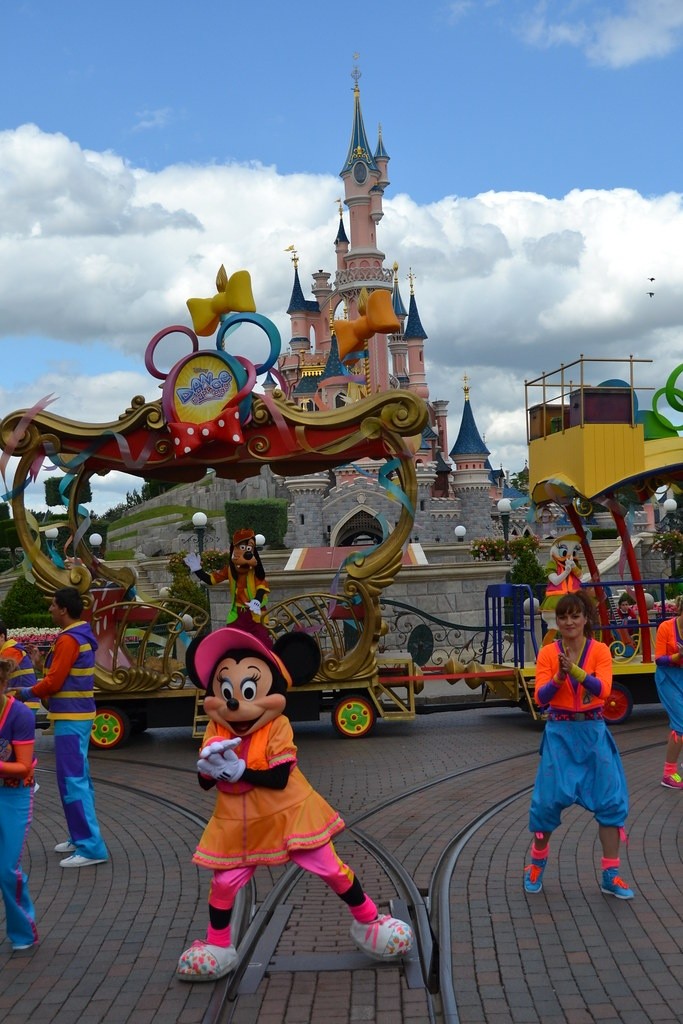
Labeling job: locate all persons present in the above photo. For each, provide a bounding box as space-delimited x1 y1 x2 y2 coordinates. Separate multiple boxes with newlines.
0 659 39 950
613 598 637 647
523 590 636 900
7 587 111 870
654 595 683 790
0 619 39 793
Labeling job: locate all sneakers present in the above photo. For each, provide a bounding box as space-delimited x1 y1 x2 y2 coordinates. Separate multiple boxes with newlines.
524 858 547 892
55 842 78 852
601 867 634 899
60 854 106 867
661 773 683 789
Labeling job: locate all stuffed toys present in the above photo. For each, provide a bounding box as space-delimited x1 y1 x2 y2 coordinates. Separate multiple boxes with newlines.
176 628 413 981
184 529 273 645
537 526 592 652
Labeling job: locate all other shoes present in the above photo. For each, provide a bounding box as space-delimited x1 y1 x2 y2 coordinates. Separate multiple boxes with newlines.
13 942 33 949
42 726 55 735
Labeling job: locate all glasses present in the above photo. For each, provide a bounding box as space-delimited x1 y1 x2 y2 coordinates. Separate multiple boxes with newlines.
622 603 629 606
